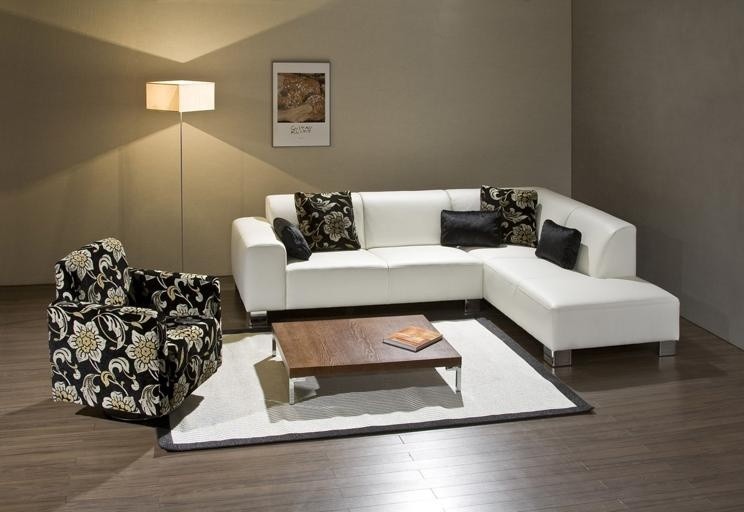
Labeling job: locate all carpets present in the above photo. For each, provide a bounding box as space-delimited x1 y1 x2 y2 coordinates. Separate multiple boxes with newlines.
157 315 595 450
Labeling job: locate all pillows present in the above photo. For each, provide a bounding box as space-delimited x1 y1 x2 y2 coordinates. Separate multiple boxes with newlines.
440 187 580 269
272 190 362 261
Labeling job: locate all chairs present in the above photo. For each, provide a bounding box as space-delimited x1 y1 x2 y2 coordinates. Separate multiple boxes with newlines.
45 237 222 421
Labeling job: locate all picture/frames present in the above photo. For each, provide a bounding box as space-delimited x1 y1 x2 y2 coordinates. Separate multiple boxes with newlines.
272 61 331 148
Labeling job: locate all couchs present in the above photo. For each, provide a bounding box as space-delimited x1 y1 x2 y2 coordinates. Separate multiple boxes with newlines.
232 186 680 368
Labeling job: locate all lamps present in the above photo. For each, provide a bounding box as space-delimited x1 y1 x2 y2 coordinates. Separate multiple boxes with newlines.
145 80 215 272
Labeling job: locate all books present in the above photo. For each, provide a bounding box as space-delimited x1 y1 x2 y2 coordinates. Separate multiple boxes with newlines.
382 324 445 353
